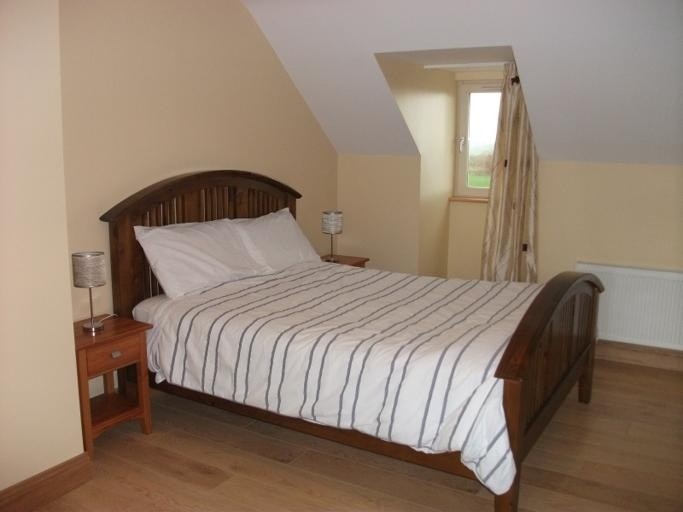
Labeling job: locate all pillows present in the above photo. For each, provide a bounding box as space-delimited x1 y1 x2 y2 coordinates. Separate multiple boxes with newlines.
130 205 320 302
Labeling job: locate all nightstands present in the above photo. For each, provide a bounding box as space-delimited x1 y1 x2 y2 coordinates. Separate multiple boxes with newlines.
320 254 372 270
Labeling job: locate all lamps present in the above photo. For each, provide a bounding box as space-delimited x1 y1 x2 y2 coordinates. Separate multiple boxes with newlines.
318 208 344 264
73 251 105 330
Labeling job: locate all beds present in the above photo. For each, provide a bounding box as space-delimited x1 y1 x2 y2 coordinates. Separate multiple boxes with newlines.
97 171 604 512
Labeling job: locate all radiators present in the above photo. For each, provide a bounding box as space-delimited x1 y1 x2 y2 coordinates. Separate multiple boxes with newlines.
575 261 682 356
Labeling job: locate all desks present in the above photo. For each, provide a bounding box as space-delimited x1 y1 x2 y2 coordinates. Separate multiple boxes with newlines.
74 314 154 454
